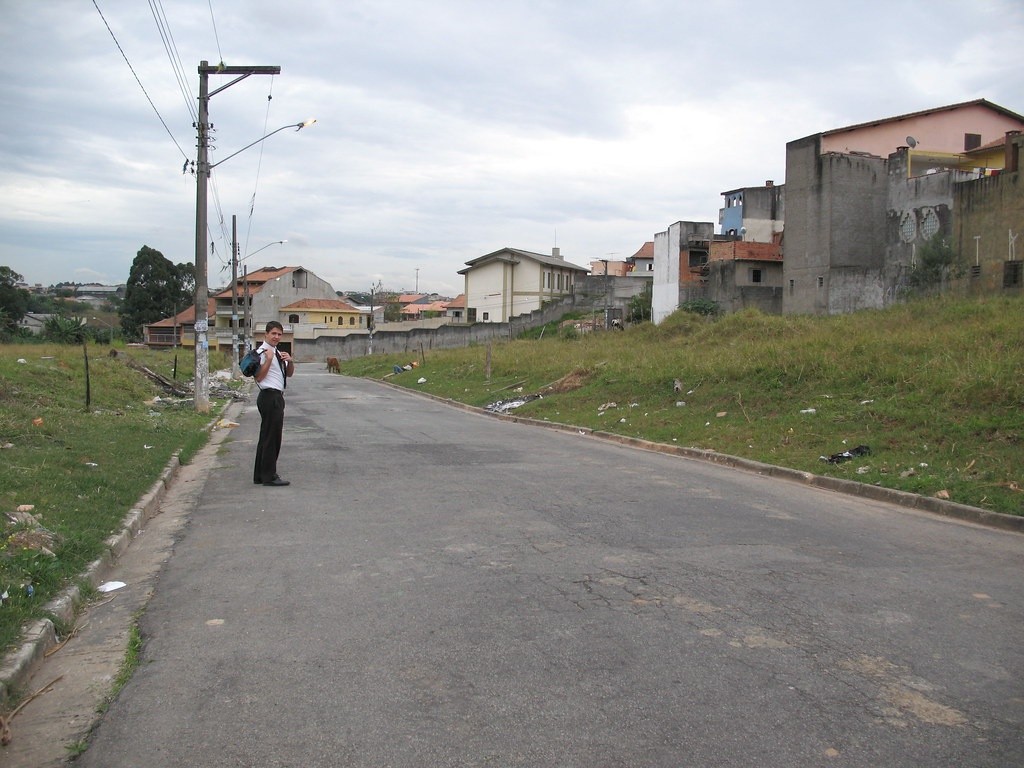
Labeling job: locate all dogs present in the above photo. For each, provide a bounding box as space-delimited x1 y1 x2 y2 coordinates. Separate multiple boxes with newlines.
326 356 340 373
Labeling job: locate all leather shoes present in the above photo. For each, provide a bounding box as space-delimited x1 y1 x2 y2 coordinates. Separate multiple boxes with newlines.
264 476 290 486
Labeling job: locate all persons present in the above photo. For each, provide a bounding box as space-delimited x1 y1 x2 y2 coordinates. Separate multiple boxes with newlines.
383 362 413 380
253 321 294 485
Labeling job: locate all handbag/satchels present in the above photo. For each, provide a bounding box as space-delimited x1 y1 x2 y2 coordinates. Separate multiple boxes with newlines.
240 348 267 377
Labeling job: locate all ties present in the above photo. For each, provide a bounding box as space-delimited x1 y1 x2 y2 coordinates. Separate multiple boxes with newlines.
276 349 286 389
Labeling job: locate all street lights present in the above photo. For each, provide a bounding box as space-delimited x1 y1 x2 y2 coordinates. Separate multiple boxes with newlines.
231 239 289 380
195 119 317 412
243 277 281 356
